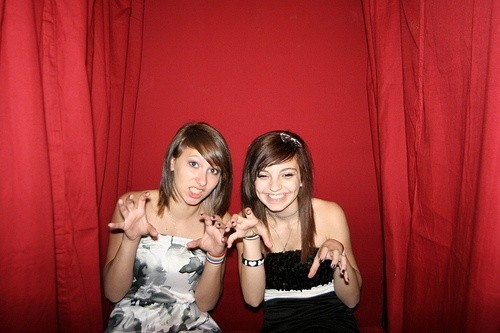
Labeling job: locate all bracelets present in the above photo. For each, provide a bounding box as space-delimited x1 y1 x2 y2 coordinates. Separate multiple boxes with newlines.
240 254 265 266
206 252 225 264
245 234 259 240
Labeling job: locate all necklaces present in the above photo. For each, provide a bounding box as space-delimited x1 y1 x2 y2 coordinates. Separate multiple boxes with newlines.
269 225 294 252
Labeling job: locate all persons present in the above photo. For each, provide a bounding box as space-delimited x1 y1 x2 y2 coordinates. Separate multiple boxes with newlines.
223 129 363 333
102 121 229 333
